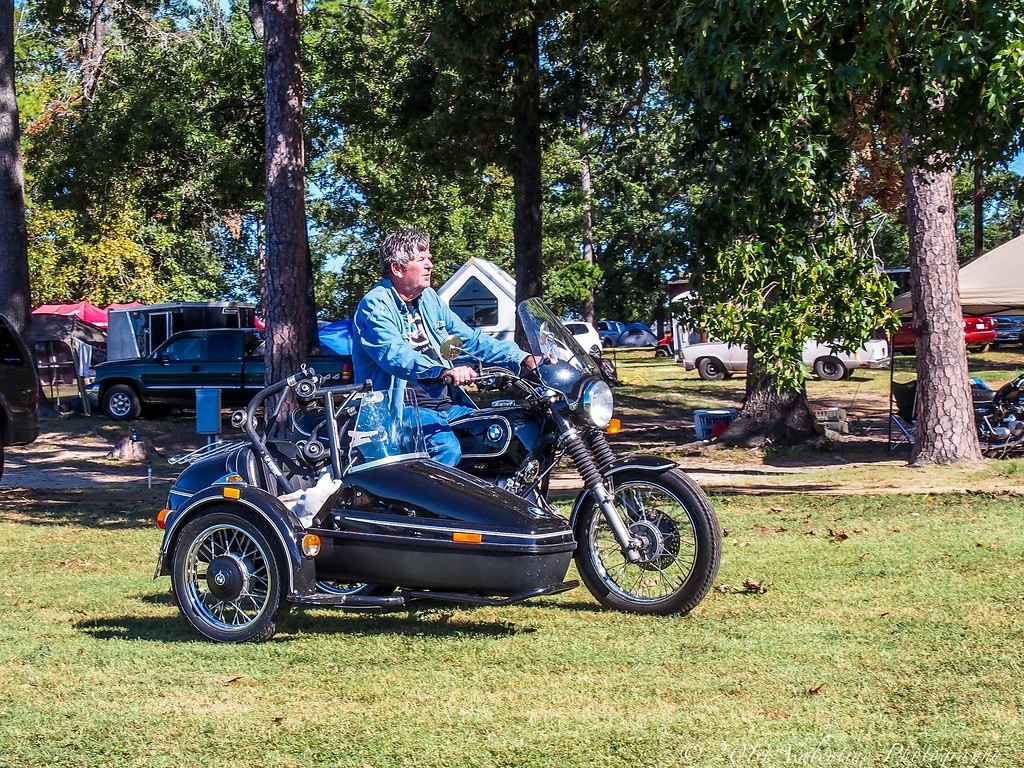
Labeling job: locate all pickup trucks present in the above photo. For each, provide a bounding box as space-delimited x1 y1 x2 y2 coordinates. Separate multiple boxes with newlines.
84 329 351 421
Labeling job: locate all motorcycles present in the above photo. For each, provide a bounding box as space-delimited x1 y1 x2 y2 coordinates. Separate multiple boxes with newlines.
154 297 721 644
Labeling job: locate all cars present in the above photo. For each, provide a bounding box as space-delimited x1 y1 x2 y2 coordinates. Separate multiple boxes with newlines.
557 321 626 361
878 270 1024 355
681 337 891 381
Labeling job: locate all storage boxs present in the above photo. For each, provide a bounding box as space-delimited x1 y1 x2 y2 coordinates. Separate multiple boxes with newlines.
692 408 738 441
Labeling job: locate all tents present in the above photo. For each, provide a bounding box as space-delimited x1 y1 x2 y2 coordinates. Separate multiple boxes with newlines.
31 301 141 417
241 318 354 358
616 323 659 347
891 234 1024 315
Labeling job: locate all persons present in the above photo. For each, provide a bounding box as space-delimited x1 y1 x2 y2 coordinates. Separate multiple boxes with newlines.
353 229 542 466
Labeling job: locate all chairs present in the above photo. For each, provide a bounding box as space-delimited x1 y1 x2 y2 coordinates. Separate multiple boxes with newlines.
888 380 919 453
